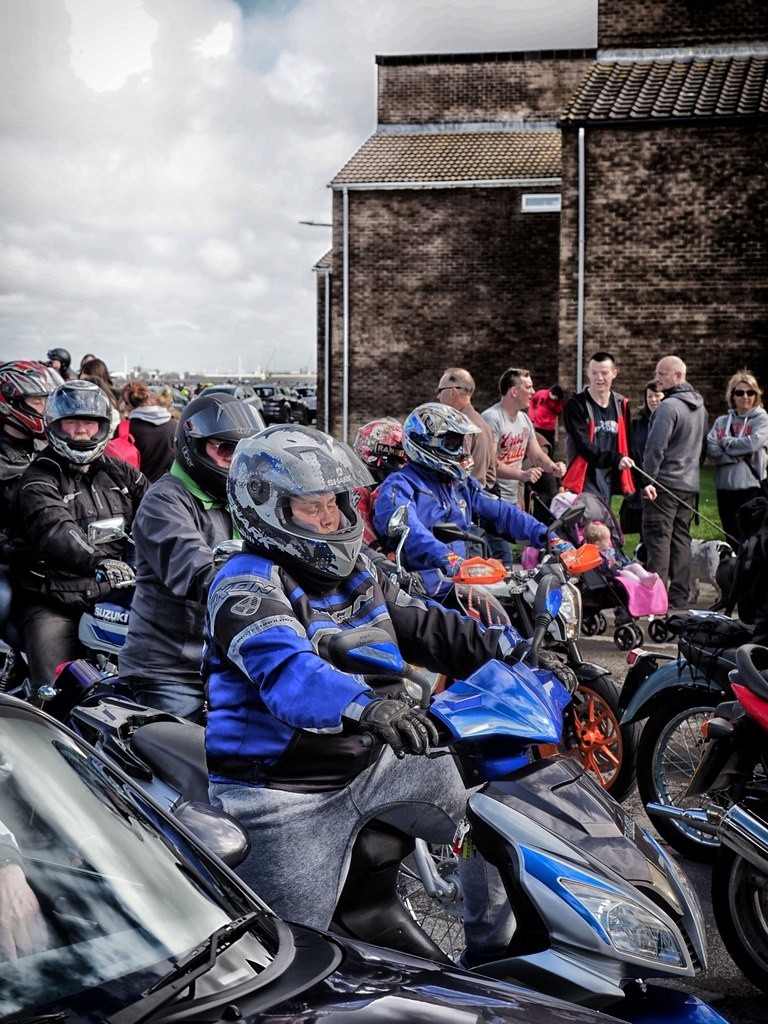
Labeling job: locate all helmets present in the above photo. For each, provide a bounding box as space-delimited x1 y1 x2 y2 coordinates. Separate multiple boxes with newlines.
43 380 112 463
403 403 482 480
227 423 365 579
0 360 61 438
48 348 71 374
354 417 407 467
173 392 259 501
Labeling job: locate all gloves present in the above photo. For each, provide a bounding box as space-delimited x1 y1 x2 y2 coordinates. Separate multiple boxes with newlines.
526 649 580 695
95 558 135 588
359 700 438 760
551 540 577 555
440 553 466 577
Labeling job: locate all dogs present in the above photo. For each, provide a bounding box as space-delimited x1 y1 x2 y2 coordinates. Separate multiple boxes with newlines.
633 533 743 618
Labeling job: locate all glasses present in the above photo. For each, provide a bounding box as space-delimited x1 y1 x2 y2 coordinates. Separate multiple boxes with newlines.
439 386 467 393
206 440 236 458
735 390 756 397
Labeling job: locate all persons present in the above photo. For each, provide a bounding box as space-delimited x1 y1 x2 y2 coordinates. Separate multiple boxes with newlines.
119 393 265 720
630 355 706 610
707 373 768 552
200 423 580 934
0 348 181 703
352 367 576 604
583 521 658 590
563 353 657 521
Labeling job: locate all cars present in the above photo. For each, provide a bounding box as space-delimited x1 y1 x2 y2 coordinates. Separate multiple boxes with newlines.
0 693 630 1023
148 385 316 424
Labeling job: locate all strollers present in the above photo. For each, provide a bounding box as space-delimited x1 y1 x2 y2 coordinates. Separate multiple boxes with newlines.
529 473 677 651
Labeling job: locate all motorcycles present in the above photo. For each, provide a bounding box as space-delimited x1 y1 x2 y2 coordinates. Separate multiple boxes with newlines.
0 519 768 1024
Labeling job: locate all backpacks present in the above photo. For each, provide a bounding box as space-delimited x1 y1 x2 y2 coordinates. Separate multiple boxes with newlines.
105 420 140 470
